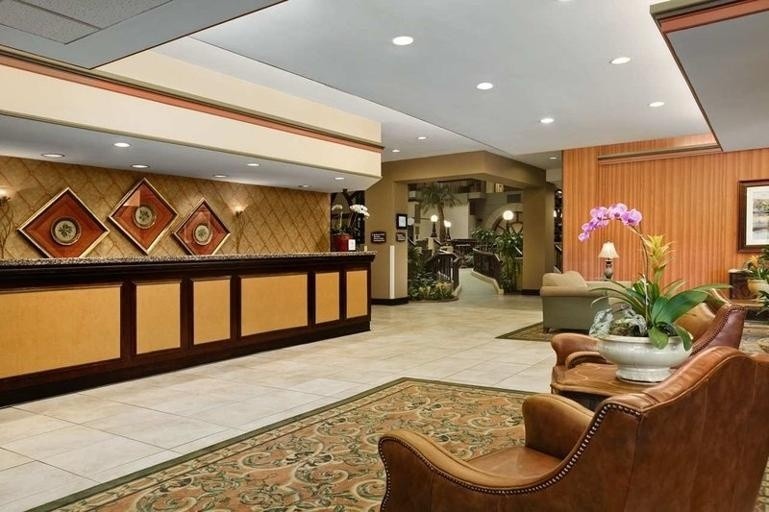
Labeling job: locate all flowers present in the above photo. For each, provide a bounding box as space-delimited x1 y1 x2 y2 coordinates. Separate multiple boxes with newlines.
577 203 733 350
345 204 369 233
742 248 769 280
331 204 344 231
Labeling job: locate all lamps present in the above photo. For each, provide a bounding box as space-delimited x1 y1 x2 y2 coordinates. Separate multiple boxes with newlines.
232 207 245 254
503 211 514 234
407 215 451 242
0 188 15 259
598 240 619 281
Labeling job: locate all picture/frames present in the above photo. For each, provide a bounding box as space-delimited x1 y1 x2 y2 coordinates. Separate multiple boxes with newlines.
371 232 386 243
738 179 769 254
17 177 232 256
396 213 407 229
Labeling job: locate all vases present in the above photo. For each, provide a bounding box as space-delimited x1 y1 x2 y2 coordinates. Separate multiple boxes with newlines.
333 232 351 252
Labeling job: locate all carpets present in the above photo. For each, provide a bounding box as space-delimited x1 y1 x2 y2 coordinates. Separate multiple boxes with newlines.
24 376 769 512
